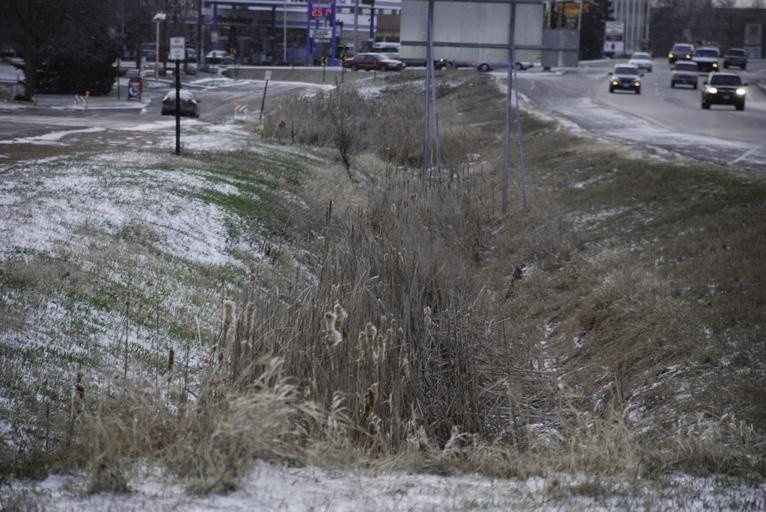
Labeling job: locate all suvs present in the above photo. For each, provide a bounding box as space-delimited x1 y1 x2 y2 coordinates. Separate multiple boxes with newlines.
691 46 719 72
668 42 694 63
670 60 699 89
722 48 747 70
700 71 745 113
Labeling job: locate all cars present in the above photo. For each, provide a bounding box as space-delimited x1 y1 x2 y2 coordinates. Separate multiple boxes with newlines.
628 52 652 72
607 62 644 94
341 51 406 71
160 90 201 117
